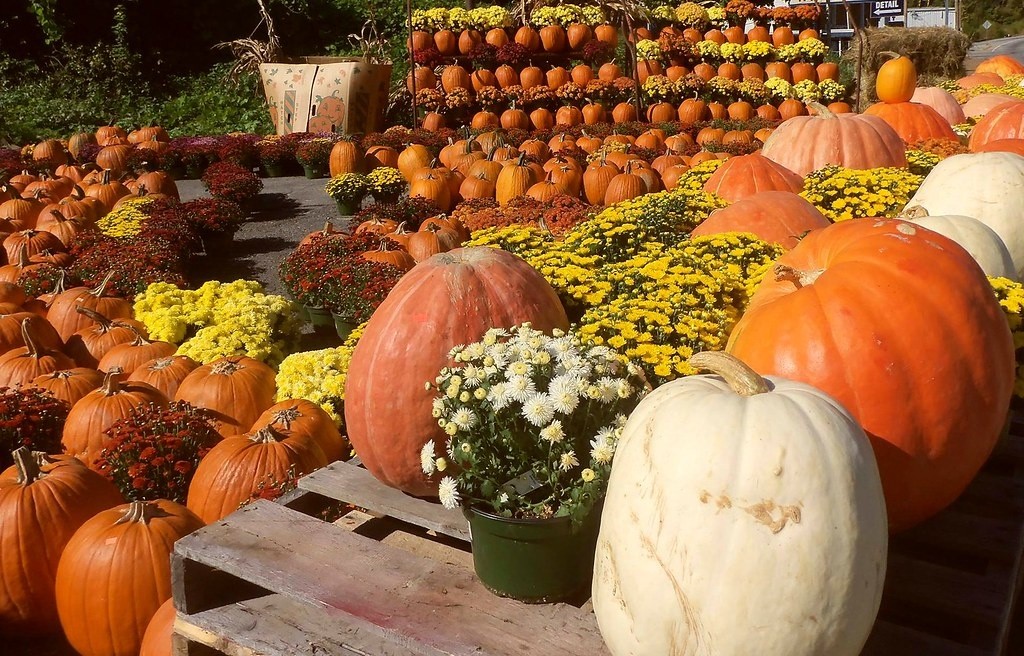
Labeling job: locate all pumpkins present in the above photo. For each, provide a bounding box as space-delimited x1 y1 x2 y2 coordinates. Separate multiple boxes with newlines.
345 243 570 500
687 50 1024 287
406 23 851 133
0 117 347 656
301 120 774 270
723 215 1014 536
591 350 887 656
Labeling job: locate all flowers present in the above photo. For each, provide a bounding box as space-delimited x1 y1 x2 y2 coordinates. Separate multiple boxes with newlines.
1 0 1024 533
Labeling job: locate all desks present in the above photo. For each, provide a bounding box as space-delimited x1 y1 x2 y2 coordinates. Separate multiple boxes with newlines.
169 459 1024 656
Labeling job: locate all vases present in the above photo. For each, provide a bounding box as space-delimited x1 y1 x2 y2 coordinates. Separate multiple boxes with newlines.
288 297 309 324
372 190 400 206
265 164 284 177
331 308 357 342
461 498 580 604
302 164 324 179
306 306 332 330
333 196 361 217
